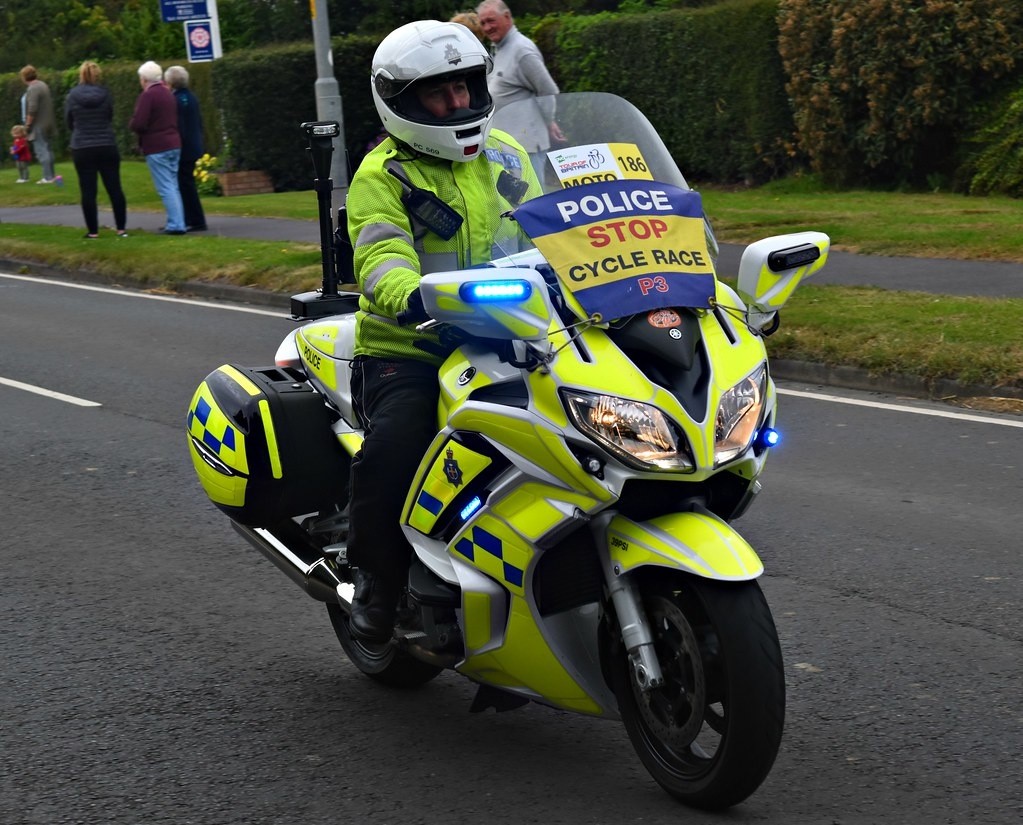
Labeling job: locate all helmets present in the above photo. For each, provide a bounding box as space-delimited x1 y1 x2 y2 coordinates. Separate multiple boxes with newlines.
370 20 496 162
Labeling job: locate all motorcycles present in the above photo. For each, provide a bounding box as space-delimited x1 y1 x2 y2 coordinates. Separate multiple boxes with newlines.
184 90 834 810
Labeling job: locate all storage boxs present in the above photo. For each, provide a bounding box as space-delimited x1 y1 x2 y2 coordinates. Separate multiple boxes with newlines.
185 361 332 527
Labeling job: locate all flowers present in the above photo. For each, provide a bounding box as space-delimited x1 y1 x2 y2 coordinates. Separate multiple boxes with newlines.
192 154 224 192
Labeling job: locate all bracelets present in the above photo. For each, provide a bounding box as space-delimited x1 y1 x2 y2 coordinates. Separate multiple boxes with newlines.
26 123 31 126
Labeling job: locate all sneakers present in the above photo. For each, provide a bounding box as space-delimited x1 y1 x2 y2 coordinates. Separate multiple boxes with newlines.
348 564 402 643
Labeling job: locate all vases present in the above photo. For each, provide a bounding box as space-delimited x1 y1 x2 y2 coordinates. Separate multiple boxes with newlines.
218 170 275 196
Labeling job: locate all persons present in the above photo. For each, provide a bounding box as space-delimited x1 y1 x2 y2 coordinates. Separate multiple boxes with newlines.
129 60 208 236
66 60 126 238
10 64 55 184
346 21 543 642
449 0 568 195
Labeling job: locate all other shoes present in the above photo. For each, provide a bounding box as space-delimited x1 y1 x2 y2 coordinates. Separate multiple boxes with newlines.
117 230 128 238
83 233 99 239
159 229 185 235
36 178 52 184
186 223 207 232
16 179 29 183
